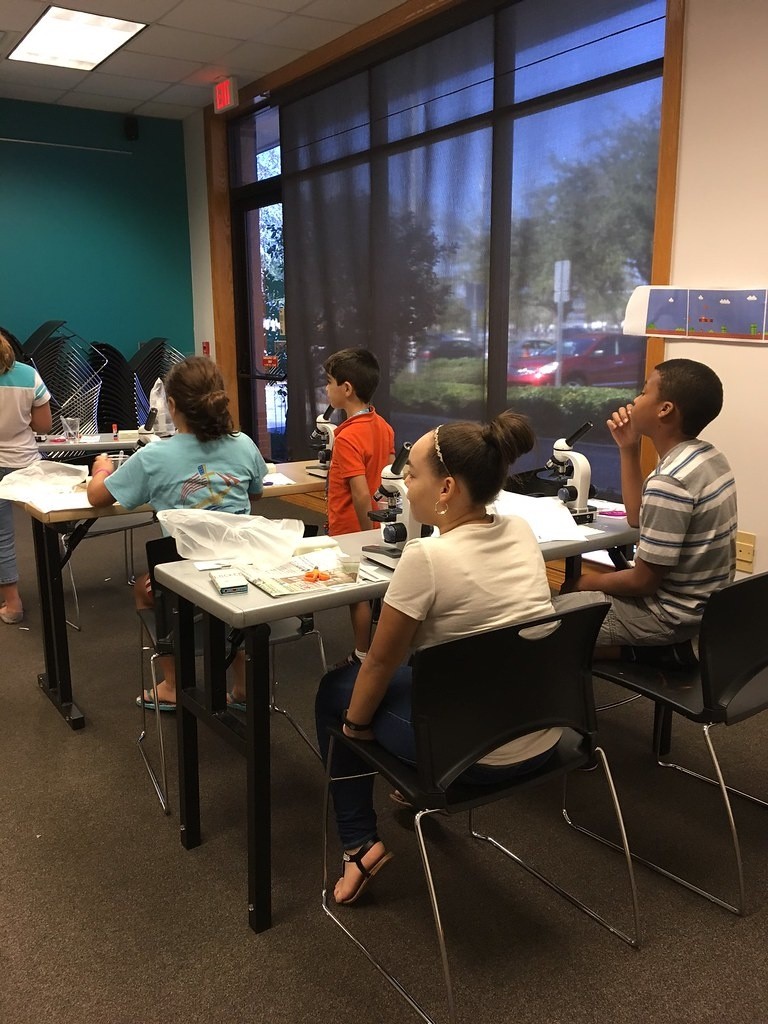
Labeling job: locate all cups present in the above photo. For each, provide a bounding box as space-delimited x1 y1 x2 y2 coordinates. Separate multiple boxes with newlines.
62 417 81 440
107 454 129 473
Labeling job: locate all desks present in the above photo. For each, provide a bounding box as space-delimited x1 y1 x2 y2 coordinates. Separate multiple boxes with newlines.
33 429 178 452
155 497 673 936
25 459 329 732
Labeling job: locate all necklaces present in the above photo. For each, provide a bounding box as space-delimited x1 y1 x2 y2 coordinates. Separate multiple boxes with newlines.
348 408 374 417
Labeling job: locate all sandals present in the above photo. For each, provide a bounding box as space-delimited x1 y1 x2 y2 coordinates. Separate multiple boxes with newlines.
333 836 395 904
389 793 413 808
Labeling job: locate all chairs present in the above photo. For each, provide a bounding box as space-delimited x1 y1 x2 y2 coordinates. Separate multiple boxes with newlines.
57 451 156 630
137 527 321 813
319 603 645 1024
560 570 768 918
20 321 187 459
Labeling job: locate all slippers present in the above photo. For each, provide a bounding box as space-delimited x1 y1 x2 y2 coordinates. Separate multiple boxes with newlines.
135 688 176 710
227 692 247 712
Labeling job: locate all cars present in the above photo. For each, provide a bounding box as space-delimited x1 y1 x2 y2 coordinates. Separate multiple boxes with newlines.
415 318 647 390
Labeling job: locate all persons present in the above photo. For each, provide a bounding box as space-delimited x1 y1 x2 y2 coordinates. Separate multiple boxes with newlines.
88 358 269 710
551 358 738 771
315 410 561 905
0 334 52 624
322 347 396 672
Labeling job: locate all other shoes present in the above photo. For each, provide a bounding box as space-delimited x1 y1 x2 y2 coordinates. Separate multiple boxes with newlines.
326 650 362 671
672 631 700 666
0 599 23 623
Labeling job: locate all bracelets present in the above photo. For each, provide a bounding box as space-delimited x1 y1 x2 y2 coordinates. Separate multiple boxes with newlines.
93 467 112 476
341 709 373 731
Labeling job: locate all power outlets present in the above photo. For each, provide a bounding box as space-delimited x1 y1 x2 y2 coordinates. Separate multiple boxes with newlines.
733 530 756 574
